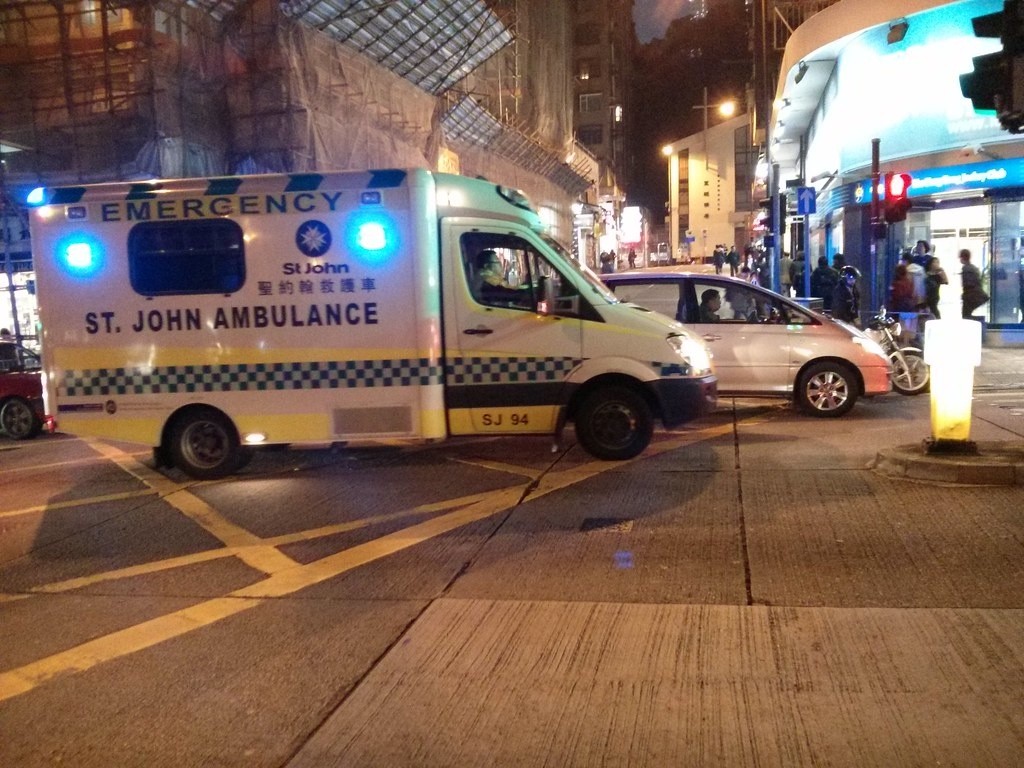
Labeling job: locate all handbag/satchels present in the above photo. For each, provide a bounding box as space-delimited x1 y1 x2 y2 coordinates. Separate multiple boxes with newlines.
966 287 989 309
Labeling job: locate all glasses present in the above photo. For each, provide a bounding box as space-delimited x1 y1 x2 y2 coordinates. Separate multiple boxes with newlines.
488 259 502 264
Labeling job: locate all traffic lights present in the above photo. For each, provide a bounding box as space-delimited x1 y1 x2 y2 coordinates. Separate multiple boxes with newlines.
885 172 913 223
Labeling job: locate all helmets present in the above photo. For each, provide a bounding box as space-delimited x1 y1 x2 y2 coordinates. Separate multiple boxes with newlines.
839 266 862 280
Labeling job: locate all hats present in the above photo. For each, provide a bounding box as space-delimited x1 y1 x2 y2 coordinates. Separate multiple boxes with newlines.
818 256 828 265
833 253 844 259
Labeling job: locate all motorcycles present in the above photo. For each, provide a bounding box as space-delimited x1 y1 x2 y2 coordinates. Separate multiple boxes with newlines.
863 305 930 396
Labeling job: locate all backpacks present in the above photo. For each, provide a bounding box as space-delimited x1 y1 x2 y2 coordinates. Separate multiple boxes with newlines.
793 261 805 290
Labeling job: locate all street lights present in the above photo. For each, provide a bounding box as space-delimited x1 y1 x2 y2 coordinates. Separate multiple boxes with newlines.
721 102 754 245
662 146 673 262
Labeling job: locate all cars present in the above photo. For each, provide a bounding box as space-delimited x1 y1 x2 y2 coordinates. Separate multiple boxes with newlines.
594 271 895 418
0 339 45 440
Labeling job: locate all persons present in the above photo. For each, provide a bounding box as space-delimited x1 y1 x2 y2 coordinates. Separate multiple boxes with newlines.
891 239 948 321
727 246 743 276
791 251 812 298
712 243 729 275
627 248 637 269
599 248 617 293
812 251 868 332
779 250 793 298
699 289 723 322
0 327 18 366
475 250 543 307
724 242 771 320
956 249 991 323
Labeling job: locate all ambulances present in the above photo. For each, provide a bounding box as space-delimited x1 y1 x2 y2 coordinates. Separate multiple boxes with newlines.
24 166 718 482
